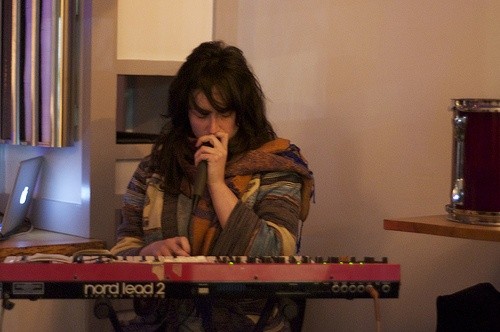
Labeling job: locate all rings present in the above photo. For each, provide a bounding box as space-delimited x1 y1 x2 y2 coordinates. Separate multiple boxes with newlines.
173 245 182 255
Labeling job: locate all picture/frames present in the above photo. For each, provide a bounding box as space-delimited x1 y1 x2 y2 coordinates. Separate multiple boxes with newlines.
447 97 500 227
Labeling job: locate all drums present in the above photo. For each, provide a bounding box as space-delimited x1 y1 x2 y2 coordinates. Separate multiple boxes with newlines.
444 98 500 226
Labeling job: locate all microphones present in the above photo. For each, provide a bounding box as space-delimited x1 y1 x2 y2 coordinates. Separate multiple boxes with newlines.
191 139 213 214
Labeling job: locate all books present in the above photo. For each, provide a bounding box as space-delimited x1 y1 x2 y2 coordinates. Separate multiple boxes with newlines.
24 249 118 263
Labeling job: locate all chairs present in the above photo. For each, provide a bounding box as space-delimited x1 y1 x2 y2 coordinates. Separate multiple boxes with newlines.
93 222 307 331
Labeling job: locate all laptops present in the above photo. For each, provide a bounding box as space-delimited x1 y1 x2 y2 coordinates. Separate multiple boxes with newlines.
0 155 44 237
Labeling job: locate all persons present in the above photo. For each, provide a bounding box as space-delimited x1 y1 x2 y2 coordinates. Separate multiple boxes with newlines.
109 38 315 332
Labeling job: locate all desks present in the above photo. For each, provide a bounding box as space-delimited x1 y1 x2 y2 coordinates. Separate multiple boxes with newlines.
384 214 500 242
0 228 107 332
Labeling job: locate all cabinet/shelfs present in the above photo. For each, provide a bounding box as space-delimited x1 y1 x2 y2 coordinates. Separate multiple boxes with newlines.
0 0 238 332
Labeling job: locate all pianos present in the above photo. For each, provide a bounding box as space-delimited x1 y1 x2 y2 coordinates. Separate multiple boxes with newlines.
0 255 400 299
